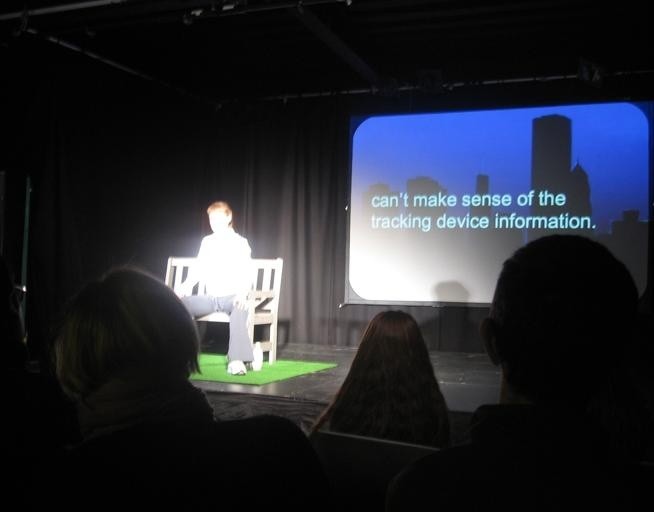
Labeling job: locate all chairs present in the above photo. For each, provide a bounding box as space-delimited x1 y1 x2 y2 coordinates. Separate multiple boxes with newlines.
0 425 654 511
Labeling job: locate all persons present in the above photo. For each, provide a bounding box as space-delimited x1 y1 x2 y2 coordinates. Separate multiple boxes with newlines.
173 201 254 375
30 266 322 512
1 266 78 512
386 233 654 512
307 309 456 512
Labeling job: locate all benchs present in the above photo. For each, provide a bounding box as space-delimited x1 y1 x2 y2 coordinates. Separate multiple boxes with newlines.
162 256 284 371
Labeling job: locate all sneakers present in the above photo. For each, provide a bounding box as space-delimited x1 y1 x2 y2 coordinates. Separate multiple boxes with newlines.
227 360 247 376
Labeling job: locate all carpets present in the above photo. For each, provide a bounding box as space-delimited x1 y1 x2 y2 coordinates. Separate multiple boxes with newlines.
188 352 338 385
